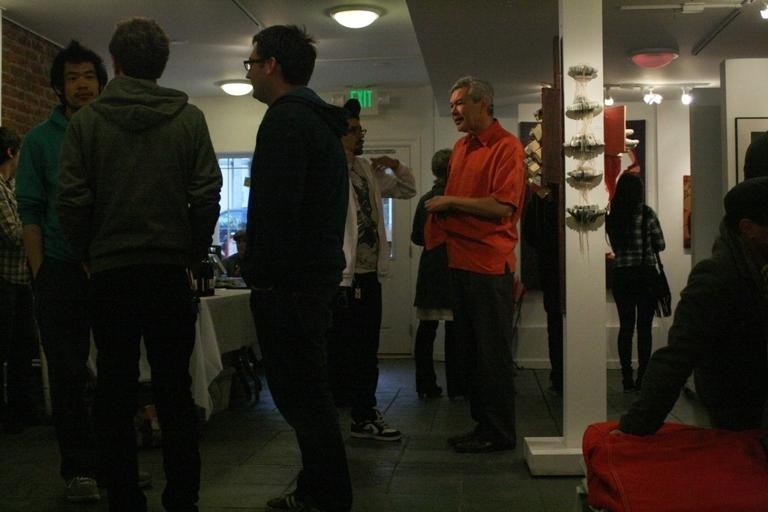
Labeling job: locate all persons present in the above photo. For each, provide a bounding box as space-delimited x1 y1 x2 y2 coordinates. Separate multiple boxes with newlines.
411 149 461 401
221 230 247 277
57 19 221 510
606 171 666 392
245 25 359 511
15 40 150 501
423 77 526 454
1 126 53 433
328 99 416 441
610 131 768 437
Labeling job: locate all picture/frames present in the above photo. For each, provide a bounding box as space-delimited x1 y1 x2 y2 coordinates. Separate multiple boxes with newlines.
734 116 768 185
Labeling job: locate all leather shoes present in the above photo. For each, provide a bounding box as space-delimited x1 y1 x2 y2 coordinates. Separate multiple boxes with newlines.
454 434 517 454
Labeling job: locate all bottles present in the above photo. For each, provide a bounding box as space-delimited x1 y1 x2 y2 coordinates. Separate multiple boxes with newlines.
192 256 215 296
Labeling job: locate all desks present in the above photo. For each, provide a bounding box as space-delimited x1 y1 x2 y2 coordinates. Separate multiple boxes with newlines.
34 288 262 421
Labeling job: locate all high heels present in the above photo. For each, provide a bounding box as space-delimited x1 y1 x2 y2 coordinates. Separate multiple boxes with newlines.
620 377 652 393
417 386 444 400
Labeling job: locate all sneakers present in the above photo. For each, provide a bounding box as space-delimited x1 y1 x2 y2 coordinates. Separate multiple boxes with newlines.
1 404 50 436
350 411 402 443
62 469 103 504
266 489 317 510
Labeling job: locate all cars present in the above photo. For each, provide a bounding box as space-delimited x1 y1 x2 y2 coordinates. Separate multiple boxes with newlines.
218 207 248 243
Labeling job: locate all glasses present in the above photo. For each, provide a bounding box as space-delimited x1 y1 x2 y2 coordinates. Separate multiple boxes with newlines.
349 127 368 136
242 57 272 70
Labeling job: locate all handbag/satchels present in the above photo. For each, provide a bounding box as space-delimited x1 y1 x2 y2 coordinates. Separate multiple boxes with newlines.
654 268 674 322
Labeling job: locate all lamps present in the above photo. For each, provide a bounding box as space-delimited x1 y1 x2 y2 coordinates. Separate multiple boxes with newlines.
599 78 710 108
214 78 256 101
323 3 384 30
